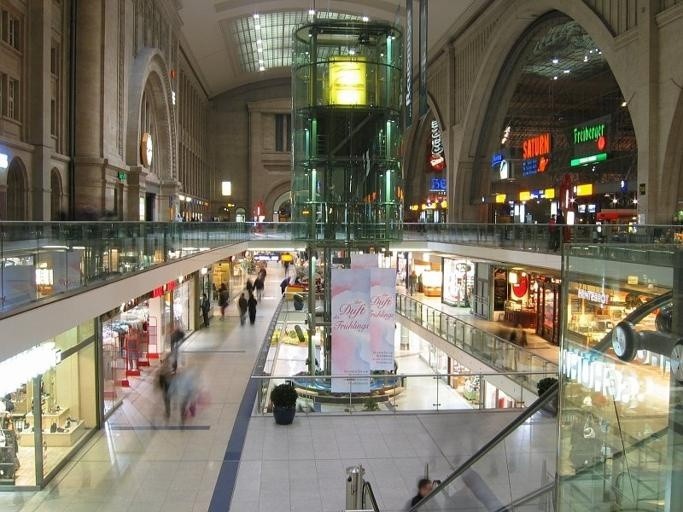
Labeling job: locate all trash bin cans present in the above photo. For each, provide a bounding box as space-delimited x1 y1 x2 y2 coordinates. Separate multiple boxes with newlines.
293 294 304 310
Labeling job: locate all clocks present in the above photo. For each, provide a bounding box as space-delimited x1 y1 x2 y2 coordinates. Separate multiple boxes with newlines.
142 133 152 167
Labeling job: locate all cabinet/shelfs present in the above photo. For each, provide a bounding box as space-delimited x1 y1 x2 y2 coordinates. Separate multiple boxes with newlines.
0 379 32 417
0 429 14 482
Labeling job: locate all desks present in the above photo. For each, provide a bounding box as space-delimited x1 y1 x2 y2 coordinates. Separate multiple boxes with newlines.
21 407 85 446
505 307 537 328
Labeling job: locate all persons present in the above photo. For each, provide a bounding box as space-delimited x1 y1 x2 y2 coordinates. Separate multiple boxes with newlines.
509 329 518 344
123 322 147 369
518 331 528 347
418 273 425 292
154 318 201 426
5 397 14 411
409 271 417 296
280 276 291 297
547 209 566 252
403 478 442 512
1 411 20 458
200 265 266 327
